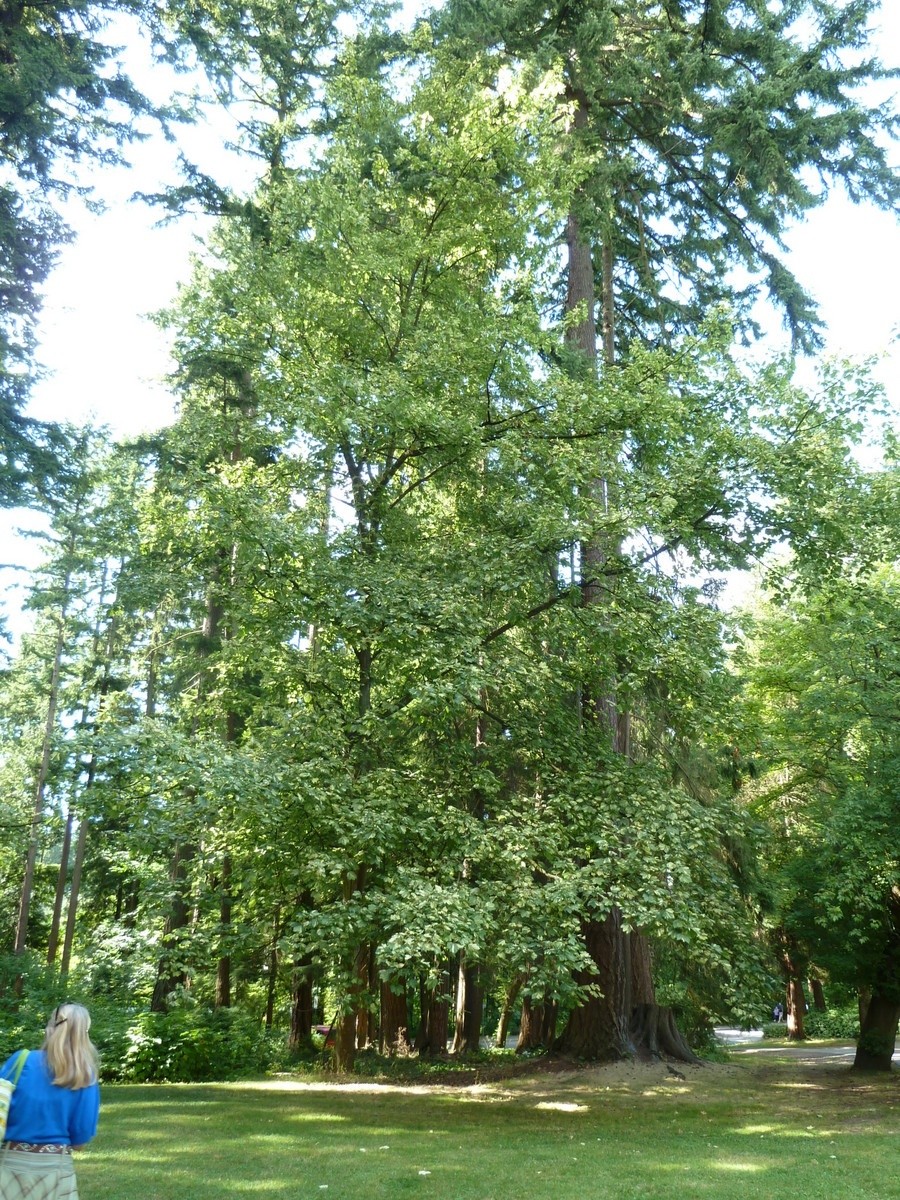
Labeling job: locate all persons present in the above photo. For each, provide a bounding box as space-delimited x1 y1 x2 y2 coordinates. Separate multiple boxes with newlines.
0 1000 101 1200
773 999 809 1022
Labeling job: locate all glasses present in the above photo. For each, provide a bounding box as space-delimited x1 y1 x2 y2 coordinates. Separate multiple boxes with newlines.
53 1002 85 1029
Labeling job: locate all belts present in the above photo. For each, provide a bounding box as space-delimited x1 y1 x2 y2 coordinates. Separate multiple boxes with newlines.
0 1141 72 1155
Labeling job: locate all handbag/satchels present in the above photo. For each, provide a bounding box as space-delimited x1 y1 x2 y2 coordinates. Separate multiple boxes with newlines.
0 1049 29 1147
